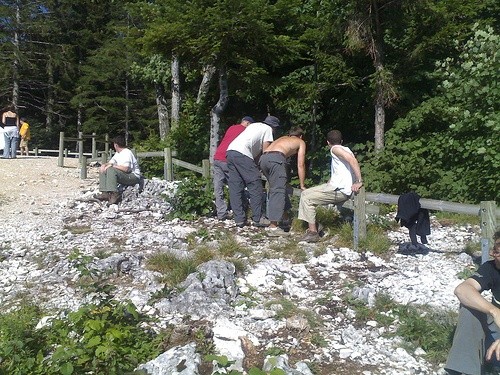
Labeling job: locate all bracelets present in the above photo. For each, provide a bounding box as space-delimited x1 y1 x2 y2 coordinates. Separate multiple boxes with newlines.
353 179 363 183
111 163 113 167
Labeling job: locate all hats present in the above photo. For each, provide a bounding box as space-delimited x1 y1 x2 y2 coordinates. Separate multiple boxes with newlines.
242 115 255 123
262 114 282 127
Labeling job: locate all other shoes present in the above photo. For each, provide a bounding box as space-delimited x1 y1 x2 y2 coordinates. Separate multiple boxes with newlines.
236 219 250 227
106 192 122 206
267 225 291 237
252 216 271 226
93 192 111 201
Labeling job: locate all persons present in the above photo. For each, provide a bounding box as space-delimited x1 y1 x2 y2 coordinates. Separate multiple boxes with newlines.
258 126 307 228
2 103 30 159
444 231 500 375
226 116 280 227
211 117 254 221
93 135 140 207
297 130 363 241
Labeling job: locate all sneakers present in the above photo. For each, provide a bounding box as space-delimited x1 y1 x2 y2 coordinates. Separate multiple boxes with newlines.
299 229 321 242
